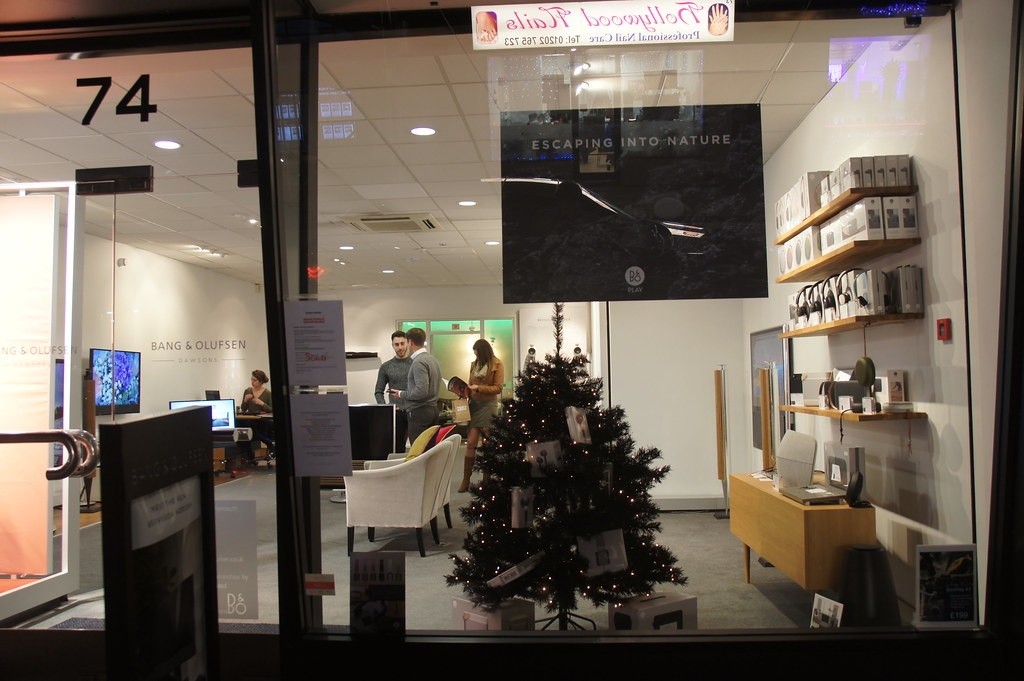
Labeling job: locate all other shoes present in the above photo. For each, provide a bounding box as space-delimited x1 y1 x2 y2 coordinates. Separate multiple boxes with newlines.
266 452 276 460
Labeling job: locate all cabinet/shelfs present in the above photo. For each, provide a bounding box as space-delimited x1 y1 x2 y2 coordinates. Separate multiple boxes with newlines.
775 155 930 422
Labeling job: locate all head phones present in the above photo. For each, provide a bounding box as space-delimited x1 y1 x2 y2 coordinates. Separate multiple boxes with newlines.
796 267 891 317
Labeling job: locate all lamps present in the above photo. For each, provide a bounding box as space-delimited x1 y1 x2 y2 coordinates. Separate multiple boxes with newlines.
469 322 475 334
489 328 495 345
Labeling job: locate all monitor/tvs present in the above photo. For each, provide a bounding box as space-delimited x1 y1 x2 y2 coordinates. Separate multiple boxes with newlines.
89 348 140 416
170 399 236 435
205 390 220 400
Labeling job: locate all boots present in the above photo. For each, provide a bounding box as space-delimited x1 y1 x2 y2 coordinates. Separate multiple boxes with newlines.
458 456 475 493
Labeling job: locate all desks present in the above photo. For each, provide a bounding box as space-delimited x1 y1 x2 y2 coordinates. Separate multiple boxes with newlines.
729 469 875 599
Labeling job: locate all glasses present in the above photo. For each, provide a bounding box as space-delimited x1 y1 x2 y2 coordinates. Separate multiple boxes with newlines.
251 378 258 381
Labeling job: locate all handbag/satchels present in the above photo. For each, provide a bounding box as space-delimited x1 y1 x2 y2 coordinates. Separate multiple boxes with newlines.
452 396 471 424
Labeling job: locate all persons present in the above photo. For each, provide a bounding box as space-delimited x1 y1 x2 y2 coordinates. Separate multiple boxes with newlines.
476 12 497 42
374 328 441 453
241 370 276 460
457 339 505 493
528 112 546 125
709 4 728 35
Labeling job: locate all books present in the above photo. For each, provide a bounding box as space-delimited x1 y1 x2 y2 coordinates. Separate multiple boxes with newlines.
440 375 469 400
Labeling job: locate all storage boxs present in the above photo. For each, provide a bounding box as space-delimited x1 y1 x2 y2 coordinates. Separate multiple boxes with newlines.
598 460 615 494
578 528 630 577
451 591 536 632
868 370 904 412
608 592 698 631
487 550 548 589
833 368 856 409
526 440 561 476
566 406 594 445
774 155 927 330
510 486 535 530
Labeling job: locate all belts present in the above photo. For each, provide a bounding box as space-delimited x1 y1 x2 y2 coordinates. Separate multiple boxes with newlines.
396 409 405 412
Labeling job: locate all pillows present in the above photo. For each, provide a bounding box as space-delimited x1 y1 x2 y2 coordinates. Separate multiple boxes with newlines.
404 425 442 463
436 425 456 445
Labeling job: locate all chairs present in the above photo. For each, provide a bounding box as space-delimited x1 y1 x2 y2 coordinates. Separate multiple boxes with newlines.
345 439 453 557
364 434 463 528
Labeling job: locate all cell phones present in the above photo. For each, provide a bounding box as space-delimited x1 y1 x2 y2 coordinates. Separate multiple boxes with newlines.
386 391 396 393
